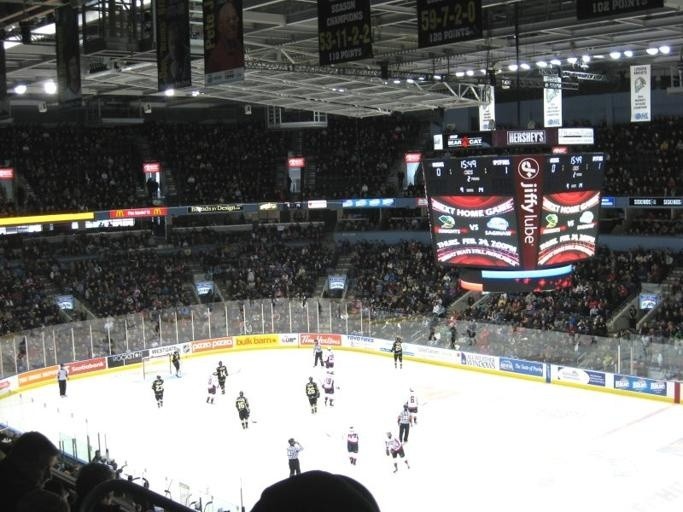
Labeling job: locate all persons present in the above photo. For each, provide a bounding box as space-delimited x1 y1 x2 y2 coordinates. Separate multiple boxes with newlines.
215 362 228 394
405 387 418 424
0 211 681 379
322 370 339 407
347 427 358 465
235 390 250 430
287 438 303 477
0 421 194 512
247 468 379 511
151 376 164 408
57 363 68 397
384 432 410 474
304 377 319 414
205 373 217 404
397 403 412 443
